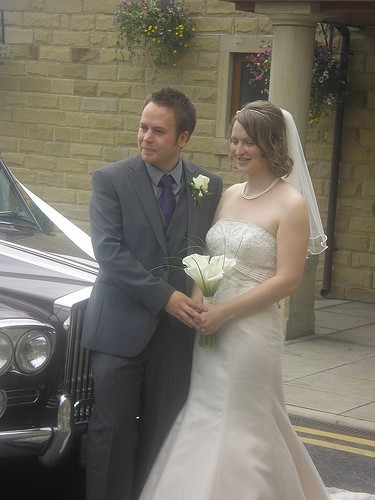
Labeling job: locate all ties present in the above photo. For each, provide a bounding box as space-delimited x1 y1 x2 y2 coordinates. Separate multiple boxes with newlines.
157 174 176 224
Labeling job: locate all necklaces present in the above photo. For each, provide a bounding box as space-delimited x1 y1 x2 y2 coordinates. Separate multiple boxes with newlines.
241 176 282 200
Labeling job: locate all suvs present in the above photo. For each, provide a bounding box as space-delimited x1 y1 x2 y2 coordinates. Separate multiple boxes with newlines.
0 156 105 470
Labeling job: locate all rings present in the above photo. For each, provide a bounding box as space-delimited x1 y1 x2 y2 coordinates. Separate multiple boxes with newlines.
202 329 207 334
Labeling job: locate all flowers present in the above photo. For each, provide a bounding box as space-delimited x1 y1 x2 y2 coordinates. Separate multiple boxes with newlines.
241 34 349 126
187 175 213 209
149 229 245 348
111 0 200 74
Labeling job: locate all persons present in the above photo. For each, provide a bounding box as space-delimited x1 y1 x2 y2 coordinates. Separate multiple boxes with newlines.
78 88 222 500
134 99 329 500
